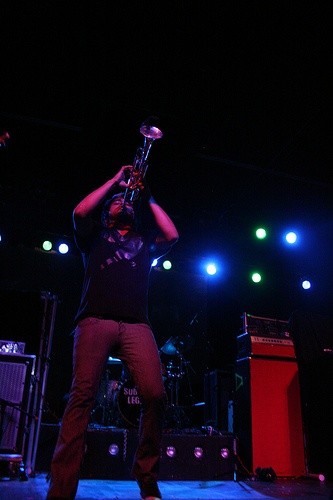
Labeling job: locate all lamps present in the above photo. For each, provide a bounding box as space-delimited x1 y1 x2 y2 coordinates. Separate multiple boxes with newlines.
285 232 297 244
41 236 73 259
162 255 176 272
250 270 263 284
256 227 269 240
302 280 312 290
204 258 221 276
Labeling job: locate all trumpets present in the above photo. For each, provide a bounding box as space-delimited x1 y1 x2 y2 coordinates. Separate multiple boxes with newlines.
121 124 163 207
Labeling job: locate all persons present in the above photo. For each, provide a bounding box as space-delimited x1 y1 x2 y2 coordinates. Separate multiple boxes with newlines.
47 165 178 500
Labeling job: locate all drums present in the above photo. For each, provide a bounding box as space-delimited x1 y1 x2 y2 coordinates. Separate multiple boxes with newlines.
96 379 120 408
113 379 169 428
161 362 184 392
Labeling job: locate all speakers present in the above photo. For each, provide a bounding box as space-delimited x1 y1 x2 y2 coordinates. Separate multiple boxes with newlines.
234 312 310 477
0 351 37 461
